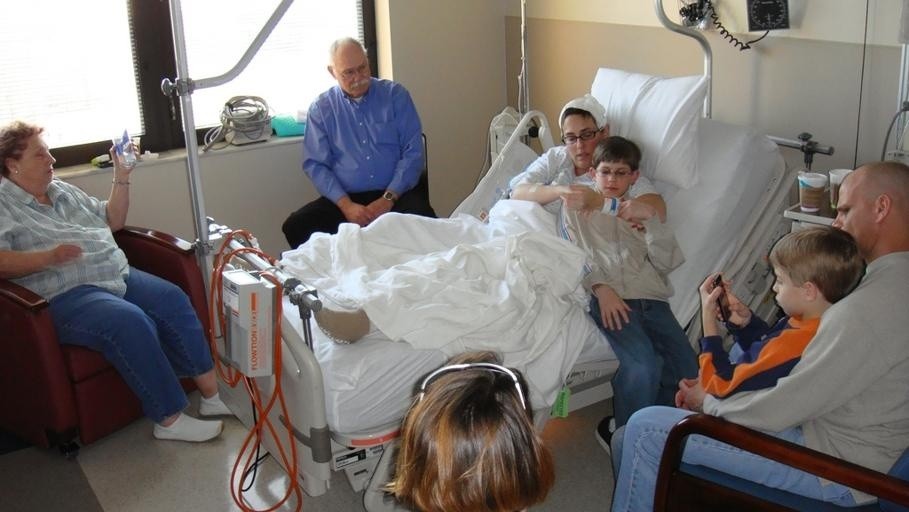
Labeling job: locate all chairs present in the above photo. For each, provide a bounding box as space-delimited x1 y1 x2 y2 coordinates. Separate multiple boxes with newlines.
419 132 430 205
653 411 908 511
0 227 214 462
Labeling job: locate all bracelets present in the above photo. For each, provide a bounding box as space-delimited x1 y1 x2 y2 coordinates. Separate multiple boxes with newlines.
112 179 131 186
602 196 622 217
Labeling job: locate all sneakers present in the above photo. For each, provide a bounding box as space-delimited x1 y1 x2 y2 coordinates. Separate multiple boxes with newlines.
199 392 232 416
594 416 617 455
153 413 223 442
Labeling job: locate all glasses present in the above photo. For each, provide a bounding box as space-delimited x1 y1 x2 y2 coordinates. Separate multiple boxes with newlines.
596 167 633 177
562 129 600 145
420 363 527 409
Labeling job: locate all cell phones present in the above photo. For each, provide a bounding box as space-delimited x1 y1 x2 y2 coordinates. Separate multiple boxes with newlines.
711 274 731 322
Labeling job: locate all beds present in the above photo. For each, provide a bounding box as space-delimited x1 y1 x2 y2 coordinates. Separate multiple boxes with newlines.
203 108 835 501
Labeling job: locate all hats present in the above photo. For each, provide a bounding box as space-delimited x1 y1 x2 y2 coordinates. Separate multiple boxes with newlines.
559 94 607 131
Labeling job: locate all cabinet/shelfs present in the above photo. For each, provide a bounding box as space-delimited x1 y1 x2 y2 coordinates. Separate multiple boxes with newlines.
784 187 837 230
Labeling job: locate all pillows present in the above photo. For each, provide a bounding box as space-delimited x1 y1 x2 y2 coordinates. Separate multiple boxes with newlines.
591 67 708 190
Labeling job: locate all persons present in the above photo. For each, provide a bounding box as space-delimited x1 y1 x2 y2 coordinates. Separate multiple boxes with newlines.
557 133 702 455
279 35 443 252
393 349 555 512
307 93 669 345
697 224 869 403
0 119 235 442
609 161 909 511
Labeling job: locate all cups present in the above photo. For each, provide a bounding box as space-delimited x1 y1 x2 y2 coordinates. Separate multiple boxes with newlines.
798 173 828 212
828 169 854 208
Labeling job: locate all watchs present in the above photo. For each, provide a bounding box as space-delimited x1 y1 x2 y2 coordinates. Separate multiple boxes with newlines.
380 190 397 205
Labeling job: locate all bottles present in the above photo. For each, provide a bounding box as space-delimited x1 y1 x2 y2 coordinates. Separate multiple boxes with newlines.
110 128 137 170
